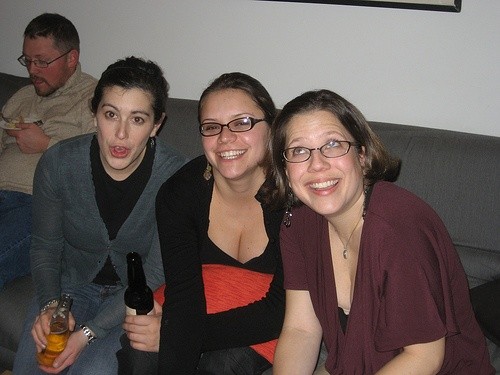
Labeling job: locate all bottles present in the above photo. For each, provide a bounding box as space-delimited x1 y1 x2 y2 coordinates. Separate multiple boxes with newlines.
35 292 72 367
124 251 156 316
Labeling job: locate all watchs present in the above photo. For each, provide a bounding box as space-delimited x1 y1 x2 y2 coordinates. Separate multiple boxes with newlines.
79 324 97 346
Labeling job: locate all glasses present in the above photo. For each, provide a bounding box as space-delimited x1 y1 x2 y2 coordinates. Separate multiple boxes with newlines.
17 50 73 69
197 115 264 137
281 138 360 164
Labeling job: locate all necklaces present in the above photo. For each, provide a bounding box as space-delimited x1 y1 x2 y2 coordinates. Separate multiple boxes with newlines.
331 216 361 260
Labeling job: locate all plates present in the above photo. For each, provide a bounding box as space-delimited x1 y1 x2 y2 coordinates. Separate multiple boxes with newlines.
0 117 38 130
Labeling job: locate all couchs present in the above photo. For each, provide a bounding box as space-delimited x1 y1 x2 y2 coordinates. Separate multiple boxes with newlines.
0 72 500 375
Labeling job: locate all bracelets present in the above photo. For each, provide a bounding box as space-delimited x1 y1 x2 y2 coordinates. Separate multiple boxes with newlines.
43 299 58 311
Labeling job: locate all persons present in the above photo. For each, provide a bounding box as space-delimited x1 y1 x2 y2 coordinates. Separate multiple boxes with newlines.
264 89 495 375
0 12 99 290
12 55 188 375
122 72 286 375
